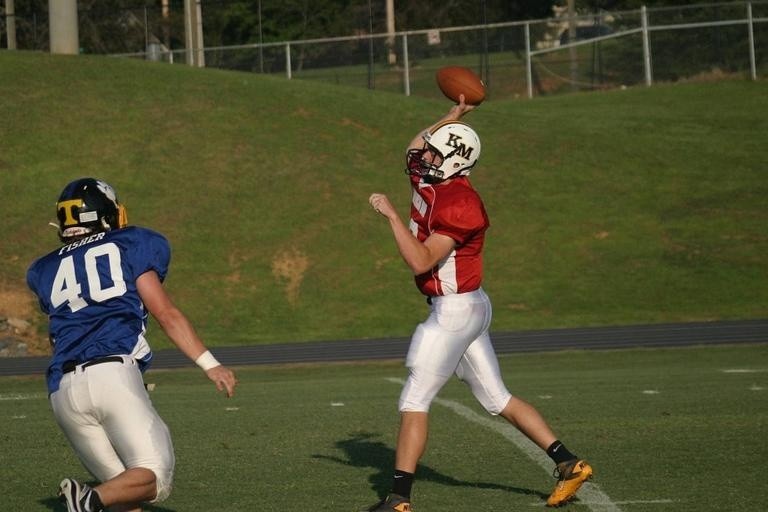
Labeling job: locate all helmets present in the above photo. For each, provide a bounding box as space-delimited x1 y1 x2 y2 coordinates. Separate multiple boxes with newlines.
404 120 481 185
54 177 129 245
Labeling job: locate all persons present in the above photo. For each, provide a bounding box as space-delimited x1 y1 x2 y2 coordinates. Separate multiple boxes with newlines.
26 178 240 510
366 93 594 511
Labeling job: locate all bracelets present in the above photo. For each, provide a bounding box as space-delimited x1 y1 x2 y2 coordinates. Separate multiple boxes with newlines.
193 349 223 374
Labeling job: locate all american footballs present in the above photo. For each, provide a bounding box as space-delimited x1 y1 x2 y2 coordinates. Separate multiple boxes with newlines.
436 67 485 104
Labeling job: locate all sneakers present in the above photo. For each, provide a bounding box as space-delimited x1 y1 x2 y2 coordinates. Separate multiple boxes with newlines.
369 489 413 511
545 455 593 508
58 476 103 511
